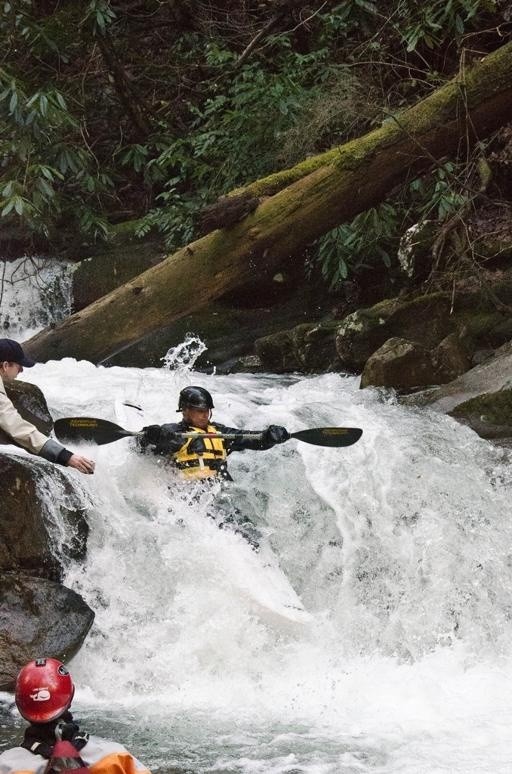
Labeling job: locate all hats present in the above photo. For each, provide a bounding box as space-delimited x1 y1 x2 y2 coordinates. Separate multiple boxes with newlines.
0 339 35 368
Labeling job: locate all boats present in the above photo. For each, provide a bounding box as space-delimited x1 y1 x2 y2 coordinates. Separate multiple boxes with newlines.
137 424 317 632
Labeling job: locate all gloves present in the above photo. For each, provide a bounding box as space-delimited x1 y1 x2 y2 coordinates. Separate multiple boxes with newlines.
267 424 289 444
143 424 174 447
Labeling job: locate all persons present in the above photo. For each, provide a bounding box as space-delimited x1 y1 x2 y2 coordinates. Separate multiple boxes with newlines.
1 655 153 774
0 338 97 476
134 383 290 557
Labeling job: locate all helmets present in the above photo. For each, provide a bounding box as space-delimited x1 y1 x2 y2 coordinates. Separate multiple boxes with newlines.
14 658 75 725
176 387 215 413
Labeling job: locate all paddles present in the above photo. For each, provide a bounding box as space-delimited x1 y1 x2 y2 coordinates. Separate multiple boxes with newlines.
54 417 363 446
